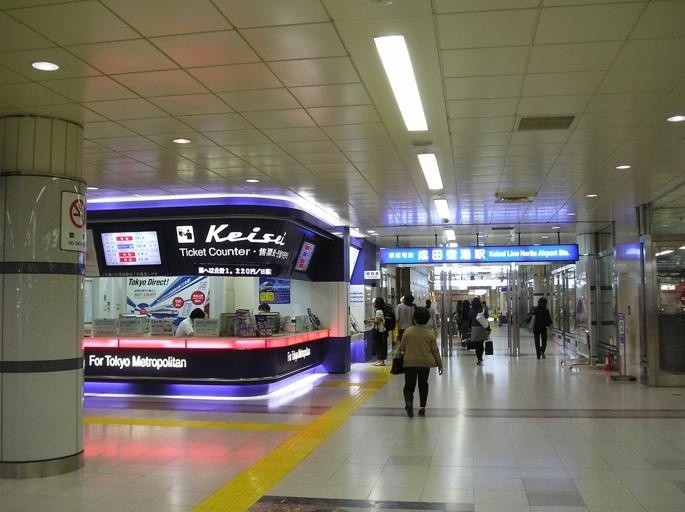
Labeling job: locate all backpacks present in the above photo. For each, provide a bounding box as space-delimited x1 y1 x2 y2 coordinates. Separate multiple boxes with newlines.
376 303 396 331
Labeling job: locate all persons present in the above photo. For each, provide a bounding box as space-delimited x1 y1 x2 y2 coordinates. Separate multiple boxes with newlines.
257 303 270 313
459 299 472 341
576 299 586 321
435 314 442 328
495 307 502 315
395 293 415 343
455 299 464 337
424 299 437 331
364 297 388 366
174 307 205 337
482 300 489 318
467 297 492 365
397 306 444 418
525 297 554 359
348 306 359 336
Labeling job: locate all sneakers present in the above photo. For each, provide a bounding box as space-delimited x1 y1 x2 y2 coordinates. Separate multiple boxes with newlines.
418 408 425 417
371 361 385 366
537 347 545 359
477 358 484 365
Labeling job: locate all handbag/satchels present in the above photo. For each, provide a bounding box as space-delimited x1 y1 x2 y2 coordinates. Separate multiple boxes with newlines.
476 307 490 329
529 307 537 329
390 349 406 376
485 339 493 355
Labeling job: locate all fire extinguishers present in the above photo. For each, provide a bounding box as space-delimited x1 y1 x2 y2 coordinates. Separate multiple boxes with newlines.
605 351 613 371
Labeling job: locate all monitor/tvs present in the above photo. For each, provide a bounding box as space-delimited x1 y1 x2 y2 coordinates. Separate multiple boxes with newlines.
295 238 317 273
101 229 163 266
